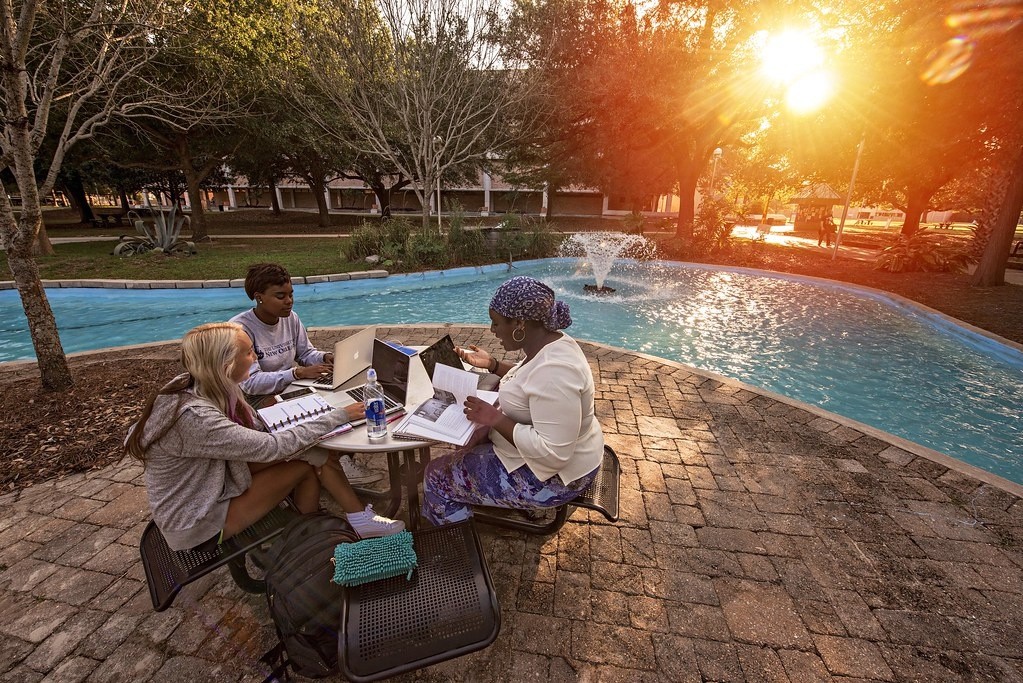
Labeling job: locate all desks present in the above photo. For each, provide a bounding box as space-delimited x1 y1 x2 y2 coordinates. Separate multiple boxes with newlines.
277 343 490 536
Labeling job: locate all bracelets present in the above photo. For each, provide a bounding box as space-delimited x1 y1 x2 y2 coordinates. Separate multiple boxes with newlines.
488 356 500 374
293 366 301 379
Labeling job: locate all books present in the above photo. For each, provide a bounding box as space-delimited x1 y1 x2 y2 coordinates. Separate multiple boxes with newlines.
390 363 498 446
257 393 353 462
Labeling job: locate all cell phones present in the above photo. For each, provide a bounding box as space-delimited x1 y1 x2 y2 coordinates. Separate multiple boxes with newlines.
274 387 318 403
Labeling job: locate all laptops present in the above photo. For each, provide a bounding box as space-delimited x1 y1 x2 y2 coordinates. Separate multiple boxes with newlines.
324 338 410 427
292 325 377 390
419 333 501 391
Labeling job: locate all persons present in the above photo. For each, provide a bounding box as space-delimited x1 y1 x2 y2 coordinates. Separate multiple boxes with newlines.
129 200 142 208
420 276 604 573
124 321 405 555
227 262 334 395
212 197 215 204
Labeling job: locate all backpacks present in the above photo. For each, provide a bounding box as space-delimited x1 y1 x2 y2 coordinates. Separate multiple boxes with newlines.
263 512 364 679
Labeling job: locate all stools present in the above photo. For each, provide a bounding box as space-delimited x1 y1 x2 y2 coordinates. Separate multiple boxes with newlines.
339 516 500 681
142 486 304 612
466 442 621 536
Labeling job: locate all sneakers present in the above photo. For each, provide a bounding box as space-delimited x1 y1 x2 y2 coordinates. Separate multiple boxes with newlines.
347 504 405 539
339 454 384 485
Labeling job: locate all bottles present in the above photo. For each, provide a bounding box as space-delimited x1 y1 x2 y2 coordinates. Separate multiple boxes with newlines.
363 369 387 437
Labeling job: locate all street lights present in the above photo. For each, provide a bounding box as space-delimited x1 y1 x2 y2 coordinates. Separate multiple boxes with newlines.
709 148 723 197
